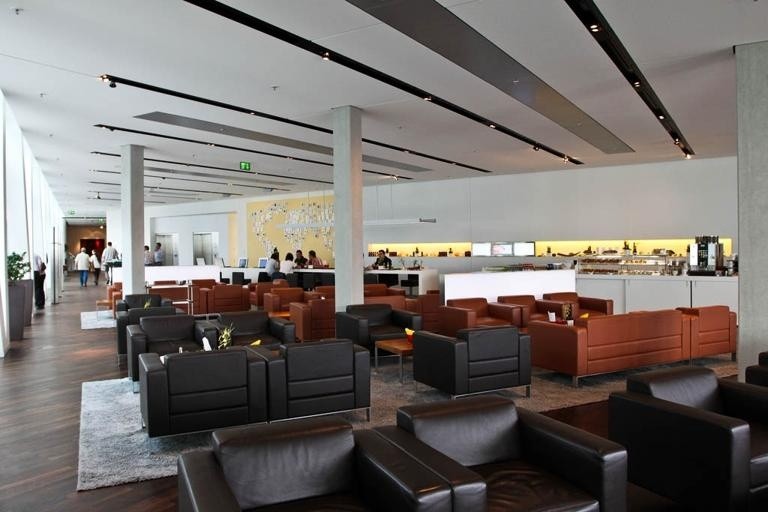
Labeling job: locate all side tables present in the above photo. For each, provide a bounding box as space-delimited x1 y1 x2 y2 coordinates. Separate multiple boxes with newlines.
94 299 112 320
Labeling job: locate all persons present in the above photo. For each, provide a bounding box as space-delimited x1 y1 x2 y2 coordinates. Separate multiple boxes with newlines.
34 252 46 310
305 250 324 268
371 250 393 269
144 246 153 265
270 247 279 263
153 243 165 264
102 241 119 284
294 250 308 268
74 247 90 287
88 249 101 286
264 252 279 273
279 253 301 275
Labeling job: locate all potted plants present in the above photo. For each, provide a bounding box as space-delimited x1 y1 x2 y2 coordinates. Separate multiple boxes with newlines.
7 250 34 342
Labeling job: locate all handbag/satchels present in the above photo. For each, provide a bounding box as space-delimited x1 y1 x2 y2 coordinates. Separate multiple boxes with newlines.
41 262 46 272
90 263 96 273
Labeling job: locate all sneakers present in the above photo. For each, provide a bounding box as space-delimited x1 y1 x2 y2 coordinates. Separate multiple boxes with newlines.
80 286 82 287
106 279 110 284
84 285 87 287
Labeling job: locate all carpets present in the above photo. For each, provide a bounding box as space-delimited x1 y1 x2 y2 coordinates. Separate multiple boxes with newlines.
79 309 117 329
76 355 739 491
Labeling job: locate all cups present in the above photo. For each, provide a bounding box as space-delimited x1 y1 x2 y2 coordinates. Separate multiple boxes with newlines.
549 313 556 322
567 320 573 326
407 335 413 344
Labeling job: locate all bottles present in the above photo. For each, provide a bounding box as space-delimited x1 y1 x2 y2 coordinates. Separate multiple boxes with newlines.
368 252 379 256
622 241 648 276
412 247 424 257
581 247 620 274
448 247 453 256
